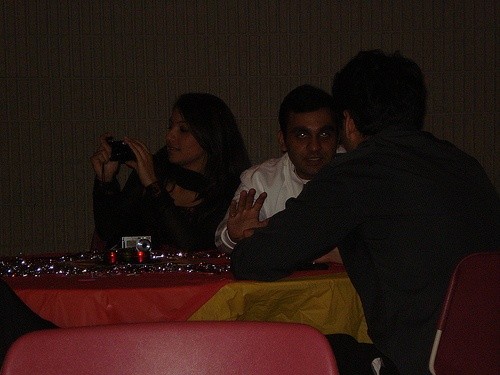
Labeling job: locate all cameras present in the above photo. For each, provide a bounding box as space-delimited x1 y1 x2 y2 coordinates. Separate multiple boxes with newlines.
106 140 137 163
122 236 151 254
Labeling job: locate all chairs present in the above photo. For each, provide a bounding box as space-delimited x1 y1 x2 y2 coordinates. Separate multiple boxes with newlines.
429 249 500 375
0 320 339 375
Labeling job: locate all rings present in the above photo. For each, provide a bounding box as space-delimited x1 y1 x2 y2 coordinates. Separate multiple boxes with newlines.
229 212 237 217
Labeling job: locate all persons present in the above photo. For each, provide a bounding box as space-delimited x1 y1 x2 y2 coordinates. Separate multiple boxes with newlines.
215 85 347 256
91 92 250 250
231 48 500 375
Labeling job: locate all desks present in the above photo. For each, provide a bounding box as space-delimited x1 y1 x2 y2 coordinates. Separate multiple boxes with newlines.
0 250 374 346
